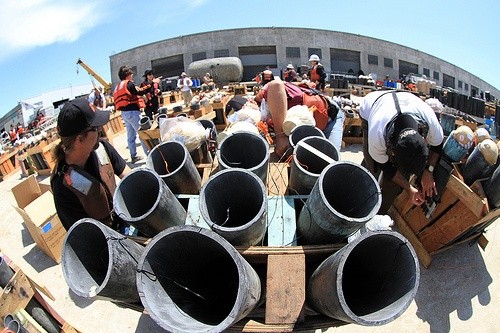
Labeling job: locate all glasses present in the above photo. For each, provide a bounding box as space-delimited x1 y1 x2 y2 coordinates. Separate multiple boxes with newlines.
80 126 98 136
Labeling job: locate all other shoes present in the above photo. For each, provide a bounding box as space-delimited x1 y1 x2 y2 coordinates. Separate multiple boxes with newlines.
132 157 143 162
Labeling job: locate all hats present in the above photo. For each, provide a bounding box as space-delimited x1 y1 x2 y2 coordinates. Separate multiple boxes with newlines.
393 114 427 173
287 64 294 69
57 99 109 137
141 70 153 77
181 72 187 76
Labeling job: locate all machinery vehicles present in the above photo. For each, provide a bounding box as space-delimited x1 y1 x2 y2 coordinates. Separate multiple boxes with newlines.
75 58 112 95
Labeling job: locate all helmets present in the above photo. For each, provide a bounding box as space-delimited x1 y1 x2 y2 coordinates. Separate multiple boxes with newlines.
309 55 320 61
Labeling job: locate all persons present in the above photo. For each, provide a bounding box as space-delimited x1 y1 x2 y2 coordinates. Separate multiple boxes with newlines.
49 99 136 238
284 63 297 82
176 72 194 106
9 112 44 144
202 71 214 90
359 90 445 214
407 77 412 84
89 85 107 136
224 66 345 158
139 68 164 119
384 74 390 81
302 55 325 92
113 65 163 162
